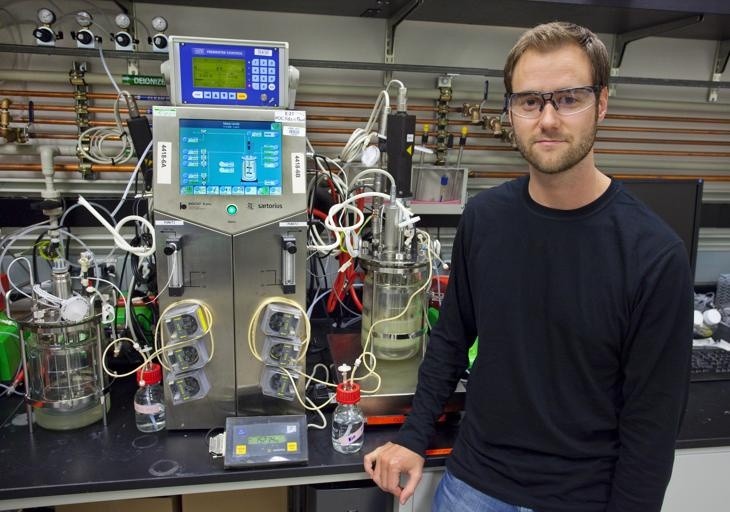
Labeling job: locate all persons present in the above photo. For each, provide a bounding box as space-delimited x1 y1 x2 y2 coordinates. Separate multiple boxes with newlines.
365 24 697 512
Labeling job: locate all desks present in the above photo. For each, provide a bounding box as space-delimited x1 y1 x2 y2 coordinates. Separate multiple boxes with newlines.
1 329 469 512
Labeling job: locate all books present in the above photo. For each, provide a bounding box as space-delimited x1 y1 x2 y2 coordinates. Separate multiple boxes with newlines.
223 414 310 468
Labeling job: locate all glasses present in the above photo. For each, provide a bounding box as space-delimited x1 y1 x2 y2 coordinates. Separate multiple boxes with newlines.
505 84 597 118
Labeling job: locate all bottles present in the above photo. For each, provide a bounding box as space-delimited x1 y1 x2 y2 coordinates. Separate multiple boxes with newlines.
134 363 165 432
331 383 367 454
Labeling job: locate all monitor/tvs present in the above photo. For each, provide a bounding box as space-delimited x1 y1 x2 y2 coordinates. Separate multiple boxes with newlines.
177 118 284 197
